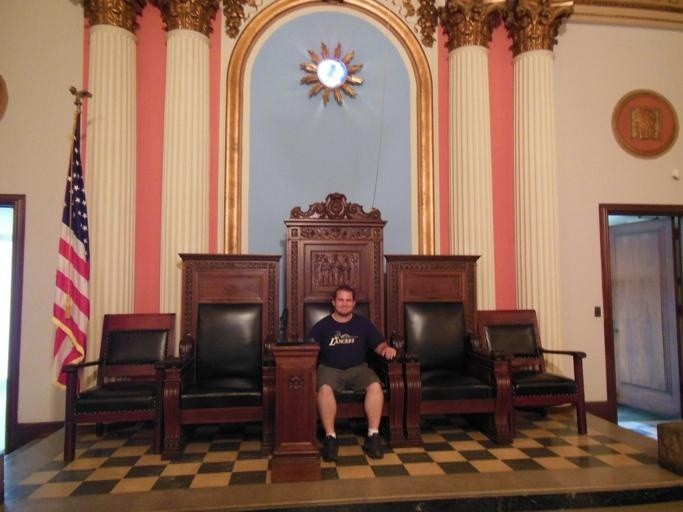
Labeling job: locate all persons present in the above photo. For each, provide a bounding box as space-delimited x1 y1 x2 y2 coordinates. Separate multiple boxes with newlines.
307 284 396 462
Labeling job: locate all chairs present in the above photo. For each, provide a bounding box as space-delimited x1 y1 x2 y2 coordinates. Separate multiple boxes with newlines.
385 254 511 448
477 309 587 437
61 313 176 462
283 192 405 448
160 253 282 461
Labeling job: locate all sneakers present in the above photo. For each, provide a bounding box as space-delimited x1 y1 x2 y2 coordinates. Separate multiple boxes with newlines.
323 435 338 461
363 433 382 457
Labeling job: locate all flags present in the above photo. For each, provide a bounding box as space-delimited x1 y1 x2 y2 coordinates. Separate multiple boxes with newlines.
52 108 92 398
